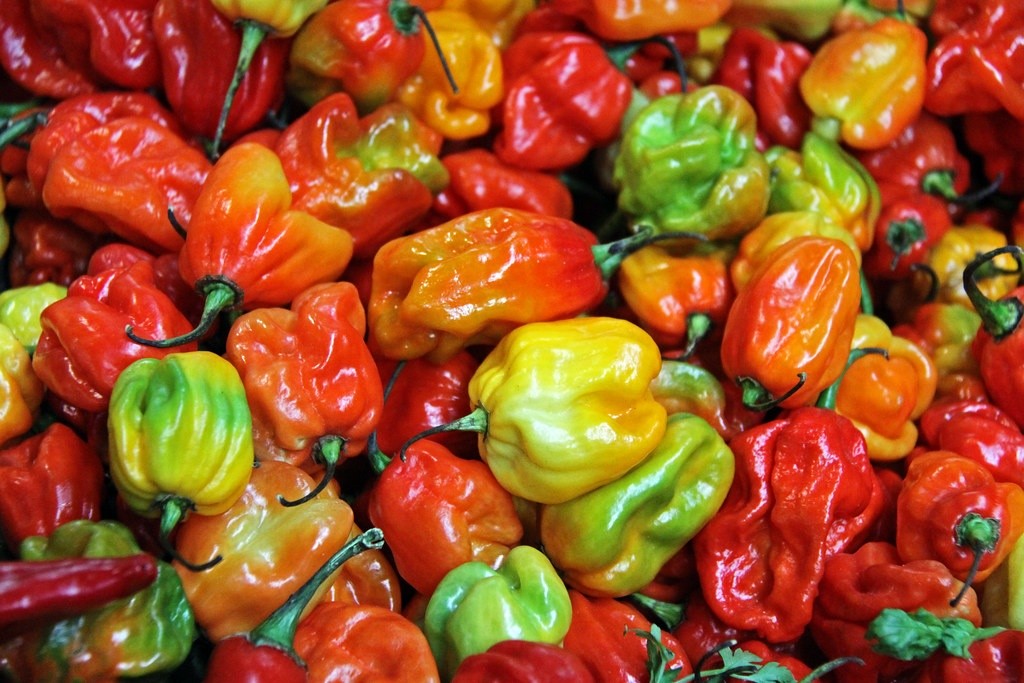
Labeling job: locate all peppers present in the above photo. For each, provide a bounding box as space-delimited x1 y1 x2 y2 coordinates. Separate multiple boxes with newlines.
0 0 1024 683
